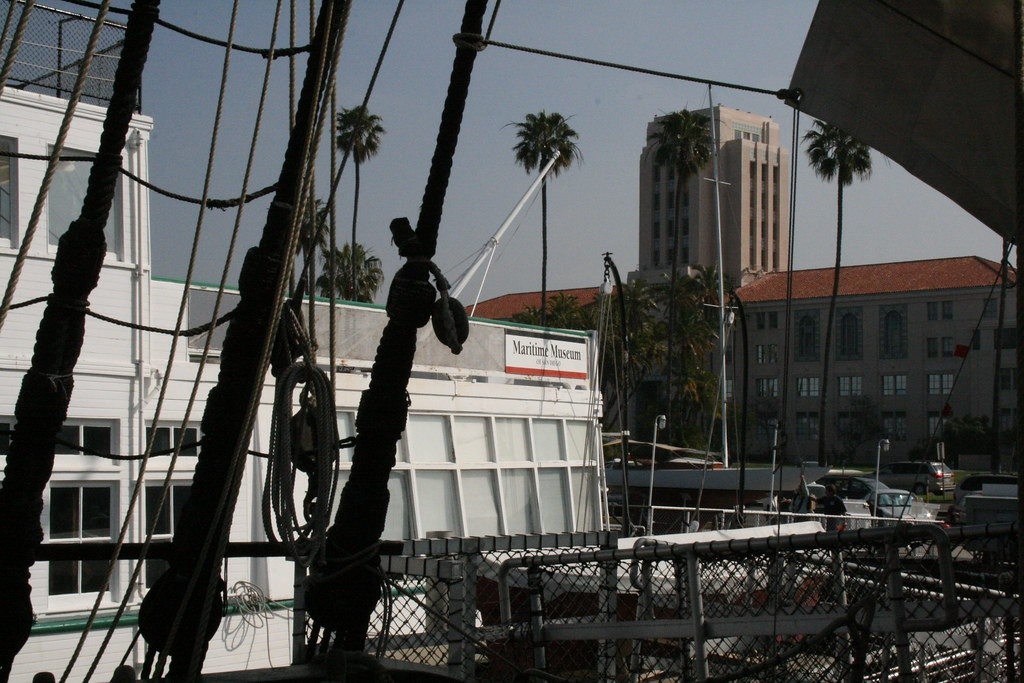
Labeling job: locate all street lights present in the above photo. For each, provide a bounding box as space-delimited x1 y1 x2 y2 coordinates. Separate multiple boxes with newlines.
873 437 891 526
648 413 667 537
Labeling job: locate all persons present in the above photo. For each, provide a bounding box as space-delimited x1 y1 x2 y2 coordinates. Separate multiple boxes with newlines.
809 484 847 531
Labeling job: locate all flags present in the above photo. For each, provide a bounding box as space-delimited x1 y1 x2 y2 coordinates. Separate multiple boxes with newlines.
941 403 953 419
954 344 970 357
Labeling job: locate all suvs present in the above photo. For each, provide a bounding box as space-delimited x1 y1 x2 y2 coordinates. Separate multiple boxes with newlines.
953 471 1018 506
862 460 957 495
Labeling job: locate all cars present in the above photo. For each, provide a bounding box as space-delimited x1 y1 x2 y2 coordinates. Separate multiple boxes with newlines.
807 468 892 516
867 489 936 525
